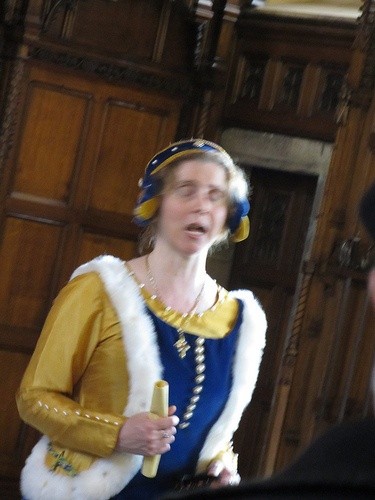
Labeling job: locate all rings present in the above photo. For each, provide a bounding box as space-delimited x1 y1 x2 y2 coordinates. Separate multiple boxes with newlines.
162 430 170 439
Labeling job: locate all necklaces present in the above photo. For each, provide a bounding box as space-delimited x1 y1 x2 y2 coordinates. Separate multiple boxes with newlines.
144 250 207 360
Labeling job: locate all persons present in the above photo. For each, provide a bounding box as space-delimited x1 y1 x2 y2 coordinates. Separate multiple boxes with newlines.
273 180 375 500
14 138 268 500
